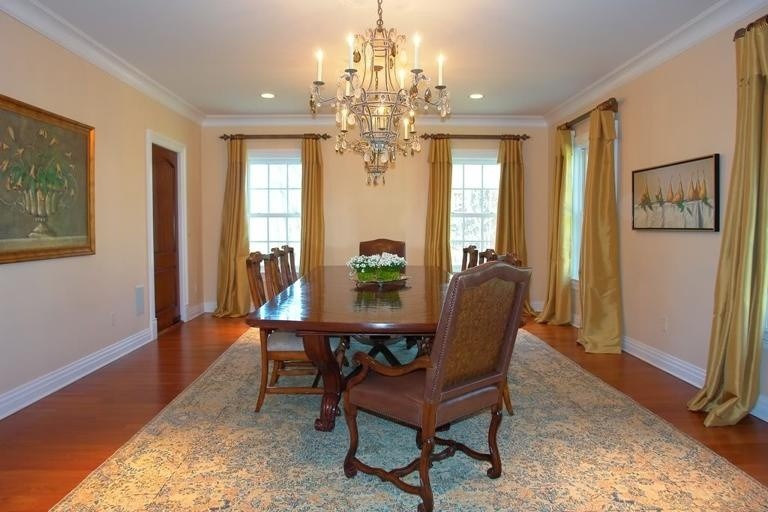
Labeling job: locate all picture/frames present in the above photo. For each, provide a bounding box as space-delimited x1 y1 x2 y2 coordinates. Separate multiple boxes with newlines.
632 153 719 232
0 94 96 265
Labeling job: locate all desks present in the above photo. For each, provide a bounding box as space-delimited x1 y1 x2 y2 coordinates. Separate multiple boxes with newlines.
246 265 454 432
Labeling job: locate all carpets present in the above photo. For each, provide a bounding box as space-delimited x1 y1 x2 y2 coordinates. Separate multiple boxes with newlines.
47 327 768 512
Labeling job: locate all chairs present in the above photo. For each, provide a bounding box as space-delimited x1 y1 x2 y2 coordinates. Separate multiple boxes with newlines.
360 239 406 264
461 245 518 416
345 261 533 511
246 245 337 412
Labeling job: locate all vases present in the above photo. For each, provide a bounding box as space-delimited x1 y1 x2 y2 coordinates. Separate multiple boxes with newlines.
345 252 408 291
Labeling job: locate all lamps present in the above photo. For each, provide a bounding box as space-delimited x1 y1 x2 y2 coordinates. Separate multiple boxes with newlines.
310 1 451 187
